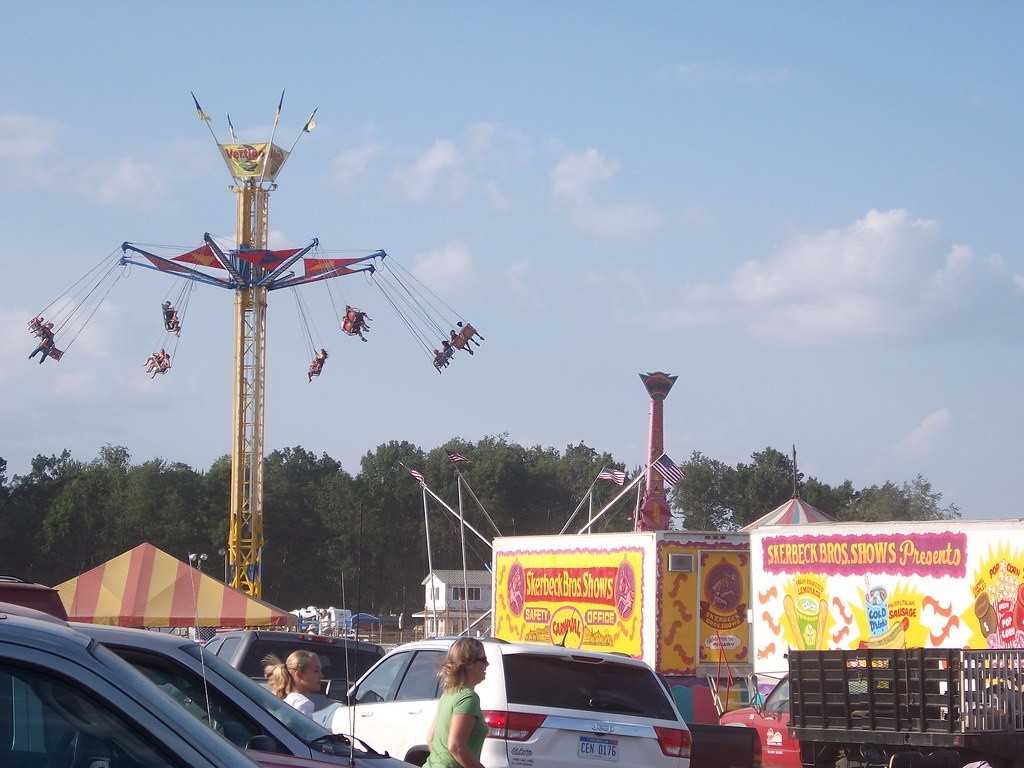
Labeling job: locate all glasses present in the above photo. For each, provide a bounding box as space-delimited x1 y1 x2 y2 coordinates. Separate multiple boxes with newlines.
471 656 488 665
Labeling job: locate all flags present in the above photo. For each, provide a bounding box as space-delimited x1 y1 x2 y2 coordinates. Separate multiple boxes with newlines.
597 467 626 486
403 464 425 483
445 450 470 463
653 453 686 486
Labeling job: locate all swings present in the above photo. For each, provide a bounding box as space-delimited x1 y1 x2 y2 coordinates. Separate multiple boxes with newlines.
381 255 477 351
149 238 208 374
27 245 127 362
310 243 363 337
369 269 456 367
288 276 328 378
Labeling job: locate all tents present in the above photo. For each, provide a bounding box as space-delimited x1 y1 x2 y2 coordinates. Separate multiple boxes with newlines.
51 542 300 635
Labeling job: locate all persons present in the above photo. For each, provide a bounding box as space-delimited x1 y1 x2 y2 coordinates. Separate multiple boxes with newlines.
28 316 56 365
450 329 474 356
342 305 373 343
163 300 181 338
147 354 170 379
433 349 447 375
442 340 454 359
307 349 328 383
262 649 325 719
457 321 485 347
421 638 490 768
142 349 165 373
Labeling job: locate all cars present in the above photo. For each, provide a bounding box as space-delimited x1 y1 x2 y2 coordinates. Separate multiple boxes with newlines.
65 621 426 768
0 614 261 768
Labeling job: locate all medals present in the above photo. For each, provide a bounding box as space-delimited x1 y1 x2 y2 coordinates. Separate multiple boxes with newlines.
346 612 381 641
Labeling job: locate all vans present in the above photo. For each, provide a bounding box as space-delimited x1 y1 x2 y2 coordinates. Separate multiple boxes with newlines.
0 574 68 622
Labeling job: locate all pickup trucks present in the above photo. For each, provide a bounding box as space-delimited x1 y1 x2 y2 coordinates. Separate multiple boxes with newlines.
717 671 807 768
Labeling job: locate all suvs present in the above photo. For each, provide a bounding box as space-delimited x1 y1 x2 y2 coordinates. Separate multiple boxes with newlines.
306 637 692 767
202 632 387 719
